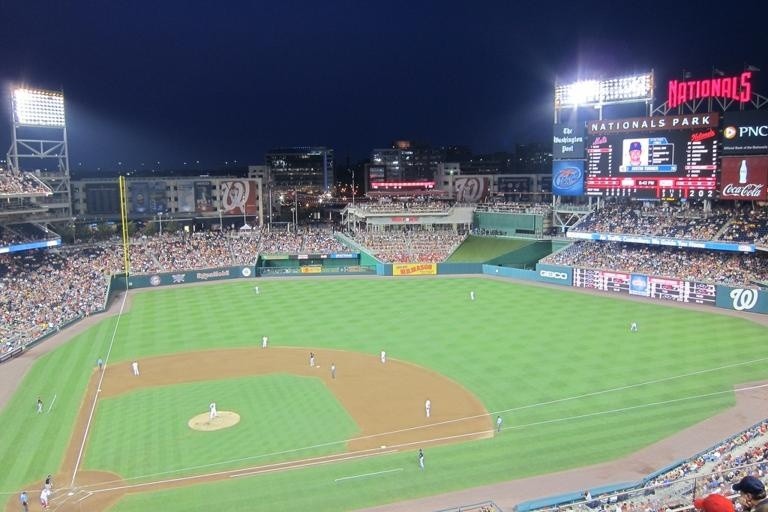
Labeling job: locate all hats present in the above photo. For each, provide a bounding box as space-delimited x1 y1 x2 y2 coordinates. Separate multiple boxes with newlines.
694 494 734 512
733 476 765 494
629 142 641 150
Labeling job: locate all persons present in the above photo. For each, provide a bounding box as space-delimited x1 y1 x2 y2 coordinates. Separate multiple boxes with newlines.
479 502 497 512
40 488 48 508
631 321 638 331
381 350 386 363
540 196 768 290
537 416 768 512
98 356 102 370
209 400 218 418
44 474 52 496
132 360 140 376
255 286 259 292
263 336 268 348
111 217 354 274
347 191 466 264
37 396 44 414
0 171 111 357
331 362 337 380
497 416 502 432
310 352 315 367
20 492 29 512
425 398 431 417
418 448 424 468
468 198 552 240
471 291 474 299
629 142 642 167
